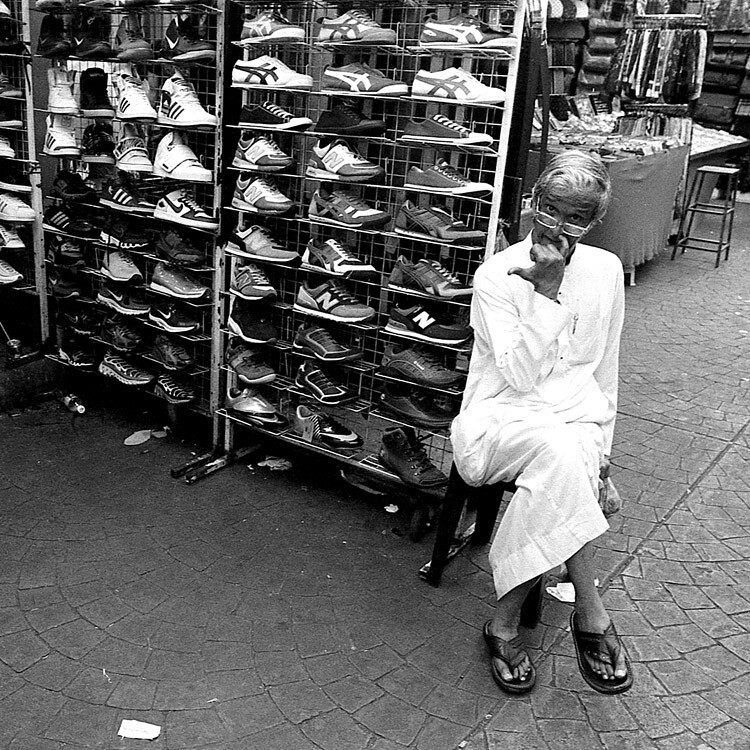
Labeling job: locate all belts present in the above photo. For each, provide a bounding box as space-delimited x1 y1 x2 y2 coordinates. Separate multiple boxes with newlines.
497 0 552 244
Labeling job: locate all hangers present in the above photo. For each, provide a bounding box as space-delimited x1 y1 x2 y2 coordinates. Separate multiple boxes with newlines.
614 101 691 120
625 18 704 38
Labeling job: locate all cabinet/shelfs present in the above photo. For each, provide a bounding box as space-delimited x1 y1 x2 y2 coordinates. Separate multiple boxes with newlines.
0 0 542 580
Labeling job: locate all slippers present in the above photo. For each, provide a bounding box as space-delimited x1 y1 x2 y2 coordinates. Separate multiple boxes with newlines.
483 618 536 693
568 607 634 695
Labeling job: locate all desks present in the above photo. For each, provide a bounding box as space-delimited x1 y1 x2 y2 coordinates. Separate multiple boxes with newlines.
522 125 690 288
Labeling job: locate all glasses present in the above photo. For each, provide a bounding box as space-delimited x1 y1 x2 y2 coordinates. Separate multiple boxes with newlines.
534 194 593 237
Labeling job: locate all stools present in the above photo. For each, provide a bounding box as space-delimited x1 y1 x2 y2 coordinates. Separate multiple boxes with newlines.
671 165 740 268
424 461 546 631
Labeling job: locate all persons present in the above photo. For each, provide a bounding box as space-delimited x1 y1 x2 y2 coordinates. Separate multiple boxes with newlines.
451 151 635 696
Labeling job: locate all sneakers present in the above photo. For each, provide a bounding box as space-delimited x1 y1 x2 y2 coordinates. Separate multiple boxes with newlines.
380 345 465 386
378 385 454 427
291 324 363 361
401 113 493 146
301 237 378 280
404 158 492 200
319 64 410 96
291 284 376 323
378 427 449 488
316 9 397 45
393 201 485 244
313 106 388 137
295 363 358 405
294 405 364 450
306 137 386 183
389 256 474 299
309 183 392 229
419 18 517 52
412 67 505 105
1 3 311 427
385 302 473 345
